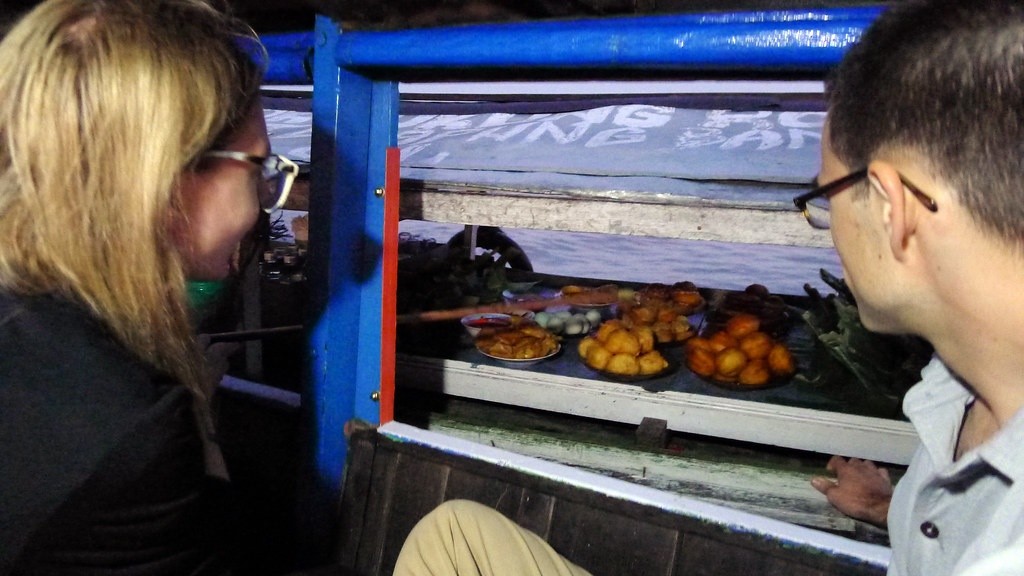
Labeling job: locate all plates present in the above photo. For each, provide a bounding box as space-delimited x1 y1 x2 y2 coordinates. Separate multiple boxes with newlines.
574 349 675 382
476 341 561 365
558 291 615 309
684 361 801 390
501 288 556 306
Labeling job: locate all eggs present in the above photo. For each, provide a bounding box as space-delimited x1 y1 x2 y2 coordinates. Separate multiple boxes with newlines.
536 309 598 335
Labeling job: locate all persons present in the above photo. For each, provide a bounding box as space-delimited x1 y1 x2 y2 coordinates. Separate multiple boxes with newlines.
0 0 306 575
389 0 1024 576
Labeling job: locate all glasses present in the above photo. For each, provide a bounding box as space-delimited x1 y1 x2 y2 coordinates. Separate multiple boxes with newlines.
792 166 937 228
206 146 300 216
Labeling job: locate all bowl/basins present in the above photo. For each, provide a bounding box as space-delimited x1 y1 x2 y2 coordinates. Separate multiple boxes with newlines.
460 313 512 338
